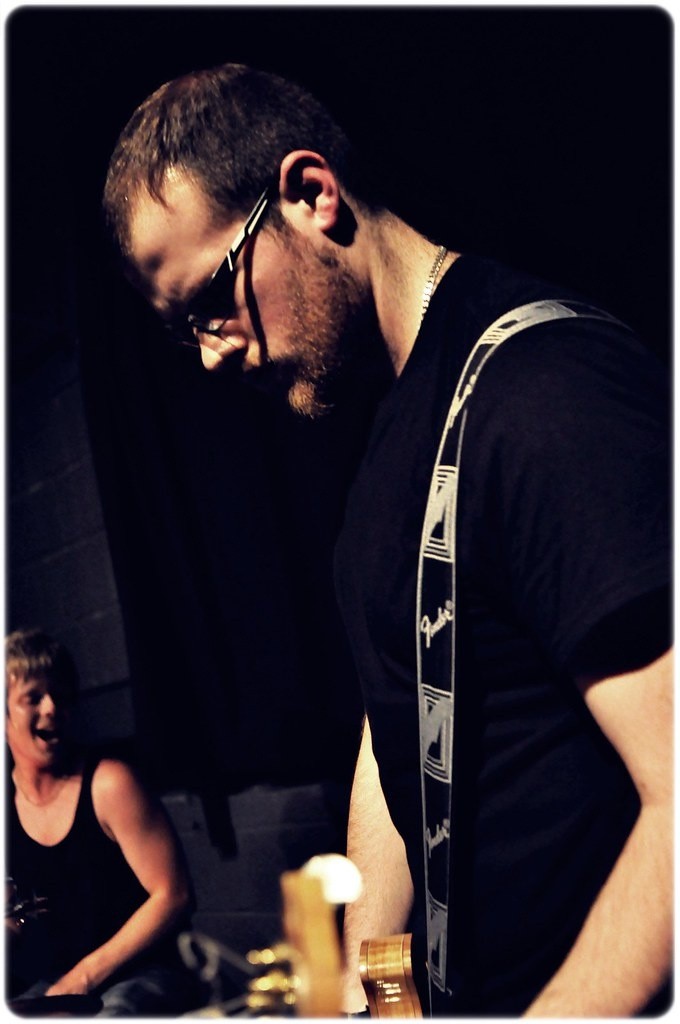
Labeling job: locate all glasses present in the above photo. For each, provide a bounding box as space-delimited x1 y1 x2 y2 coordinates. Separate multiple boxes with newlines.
166 178 278 350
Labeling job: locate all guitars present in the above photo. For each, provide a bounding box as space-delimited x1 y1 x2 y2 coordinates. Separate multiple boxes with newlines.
244 873 425 1019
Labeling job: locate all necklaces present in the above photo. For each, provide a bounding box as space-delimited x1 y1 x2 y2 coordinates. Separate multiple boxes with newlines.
19 773 68 807
422 243 449 317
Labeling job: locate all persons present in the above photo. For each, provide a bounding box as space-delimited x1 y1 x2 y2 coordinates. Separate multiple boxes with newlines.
100 61 674 1017
6 629 213 1015
